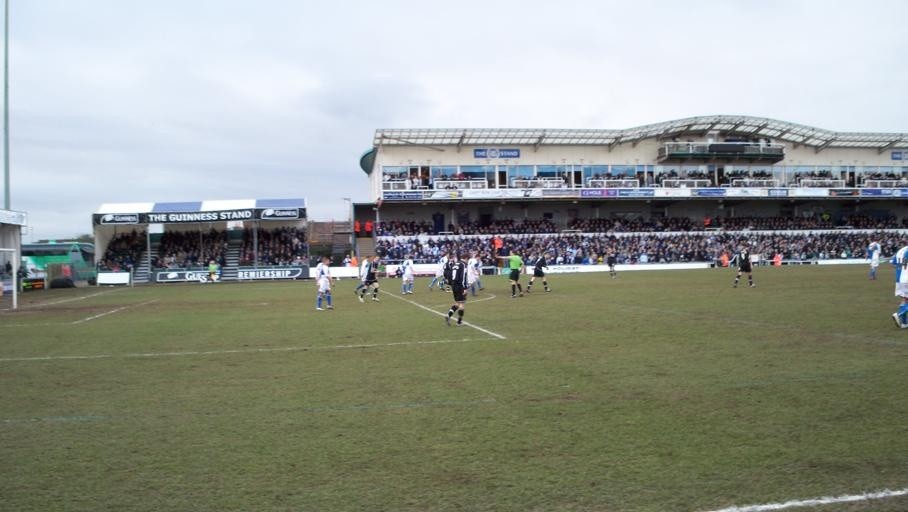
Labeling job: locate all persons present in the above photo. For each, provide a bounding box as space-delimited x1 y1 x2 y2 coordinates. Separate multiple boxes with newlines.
445 254 471 328
889 246 908 329
315 255 336 310
607 248 616 279
526 249 551 293
343 255 351 267
396 267 402 278
208 260 221 283
430 251 484 296
864 239 882 280
375 214 908 264
496 250 524 297
411 168 908 189
355 219 373 237
97 225 309 271
732 243 756 288
353 254 381 302
403 255 415 294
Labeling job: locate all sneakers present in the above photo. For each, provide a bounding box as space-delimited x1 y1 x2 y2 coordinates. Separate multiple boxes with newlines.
544 288 551 292
512 289 530 297
402 290 412 295
317 306 332 313
463 287 484 298
893 313 908 328
354 289 379 303
429 286 452 292
444 316 465 327
